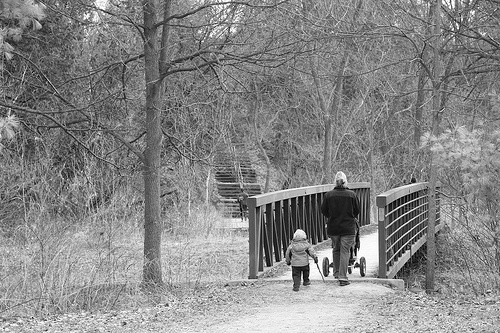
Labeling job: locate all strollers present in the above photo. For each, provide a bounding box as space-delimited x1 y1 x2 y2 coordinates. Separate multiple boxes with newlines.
322 218 366 277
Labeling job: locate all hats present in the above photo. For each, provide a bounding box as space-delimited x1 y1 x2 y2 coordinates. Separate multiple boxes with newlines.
293 229 307 239
335 171 347 187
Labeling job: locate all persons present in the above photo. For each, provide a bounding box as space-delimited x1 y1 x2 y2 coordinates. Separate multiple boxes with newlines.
238 188 249 222
321 171 360 286
408 177 417 184
285 229 318 292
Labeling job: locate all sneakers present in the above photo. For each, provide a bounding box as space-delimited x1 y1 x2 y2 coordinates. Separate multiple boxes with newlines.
340 281 351 285
293 287 299 291
303 281 311 285
